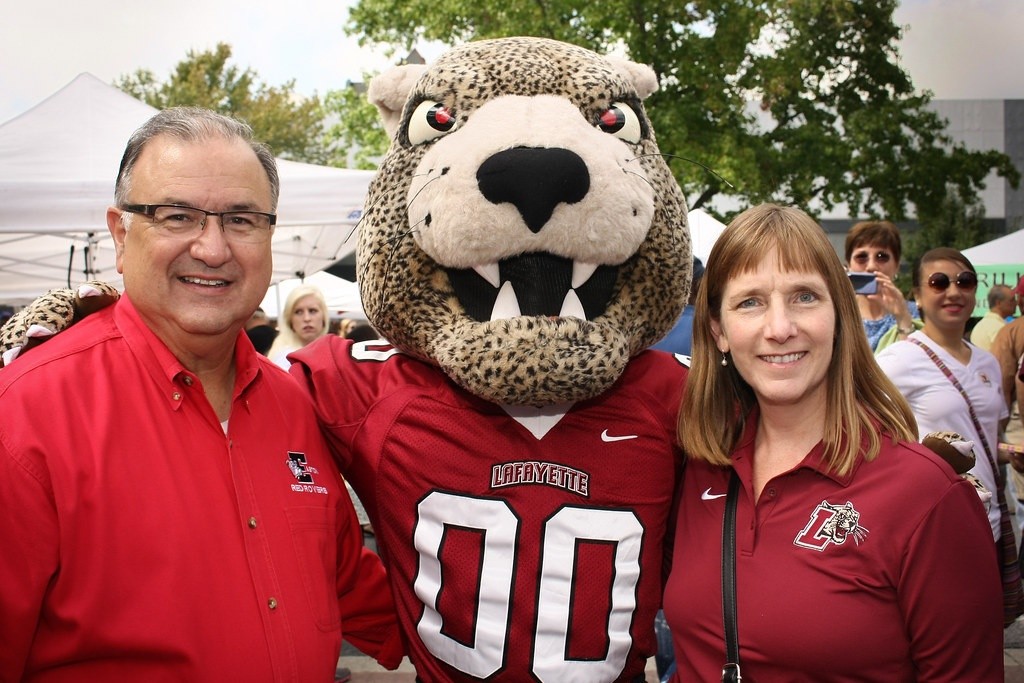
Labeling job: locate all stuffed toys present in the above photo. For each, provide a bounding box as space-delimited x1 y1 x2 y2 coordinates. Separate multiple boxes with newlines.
0 36 992 683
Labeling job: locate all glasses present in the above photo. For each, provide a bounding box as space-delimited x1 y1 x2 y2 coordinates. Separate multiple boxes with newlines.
917 269 977 294
119 202 276 243
852 250 890 264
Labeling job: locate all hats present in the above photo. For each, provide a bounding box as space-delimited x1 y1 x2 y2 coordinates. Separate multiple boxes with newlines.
693 256 705 280
340 317 352 333
1014 276 1024 297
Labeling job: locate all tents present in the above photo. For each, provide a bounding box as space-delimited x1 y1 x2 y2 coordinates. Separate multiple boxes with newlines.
0 70 380 322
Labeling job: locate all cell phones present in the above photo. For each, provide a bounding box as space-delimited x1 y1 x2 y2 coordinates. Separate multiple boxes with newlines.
842 272 879 295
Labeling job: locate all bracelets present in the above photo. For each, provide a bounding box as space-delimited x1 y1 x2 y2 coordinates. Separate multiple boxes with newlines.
896 324 915 333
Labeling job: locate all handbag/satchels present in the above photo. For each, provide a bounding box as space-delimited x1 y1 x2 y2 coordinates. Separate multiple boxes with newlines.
992 511 1024 630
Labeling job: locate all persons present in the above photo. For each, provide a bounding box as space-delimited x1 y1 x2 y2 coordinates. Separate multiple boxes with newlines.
239 288 381 372
646 256 704 365
0 107 408 683
969 275 1024 426
842 220 925 353
875 246 1024 628
662 202 1003 683
